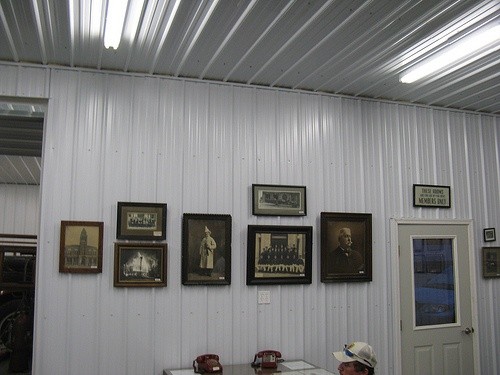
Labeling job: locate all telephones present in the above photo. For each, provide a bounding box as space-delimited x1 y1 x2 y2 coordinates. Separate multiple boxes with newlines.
251 350 281 369
192 354 223 374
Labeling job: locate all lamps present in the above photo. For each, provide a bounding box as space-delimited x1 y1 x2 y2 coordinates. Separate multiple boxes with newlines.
398 16 500 84
102 0 129 50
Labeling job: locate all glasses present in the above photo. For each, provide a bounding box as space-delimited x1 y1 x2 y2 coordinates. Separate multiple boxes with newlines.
342 344 373 366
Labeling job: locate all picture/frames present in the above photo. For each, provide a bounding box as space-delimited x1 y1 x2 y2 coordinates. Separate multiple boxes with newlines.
181 213 232 285
251 183 307 216
59 220 104 274
246 225 313 285
482 247 500 279
114 242 167 288
115 202 167 241
413 183 451 208
483 228 496 242
320 212 372 283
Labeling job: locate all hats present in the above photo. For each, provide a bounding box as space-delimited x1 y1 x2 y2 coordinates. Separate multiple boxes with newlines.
332 341 377 368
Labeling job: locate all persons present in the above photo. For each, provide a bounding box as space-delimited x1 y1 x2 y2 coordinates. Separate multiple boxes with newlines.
255 243 304 273
200 225 216 276
128 213 155 227
331 226 353 258
332 342 378 375
